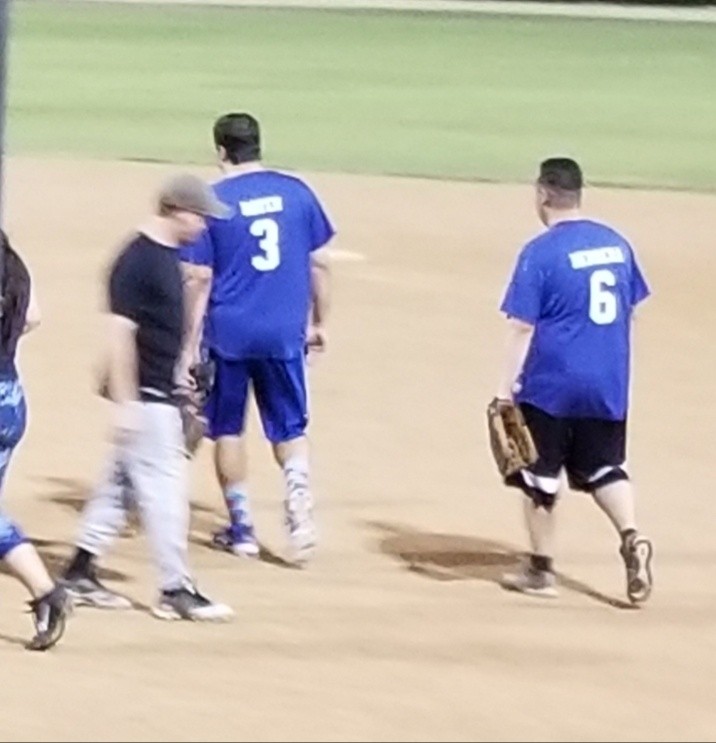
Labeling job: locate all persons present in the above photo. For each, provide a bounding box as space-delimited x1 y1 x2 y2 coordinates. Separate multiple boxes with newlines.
172 114 336 563
497 158 653 603
1 229 70 652
59 177 240 624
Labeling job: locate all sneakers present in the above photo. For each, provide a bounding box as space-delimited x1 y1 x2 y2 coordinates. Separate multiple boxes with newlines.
503 560 556 595
24 580 73 650
214 527 260 554
287 495 316 564
152 588 235 621
55 574 132 610
620 532 653 601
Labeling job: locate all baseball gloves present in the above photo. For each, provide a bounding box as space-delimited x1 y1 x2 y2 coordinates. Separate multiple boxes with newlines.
180 358 217 461
486 396 540 478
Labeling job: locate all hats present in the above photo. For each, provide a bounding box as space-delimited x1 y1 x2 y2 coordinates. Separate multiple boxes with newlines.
161 174 235 220
